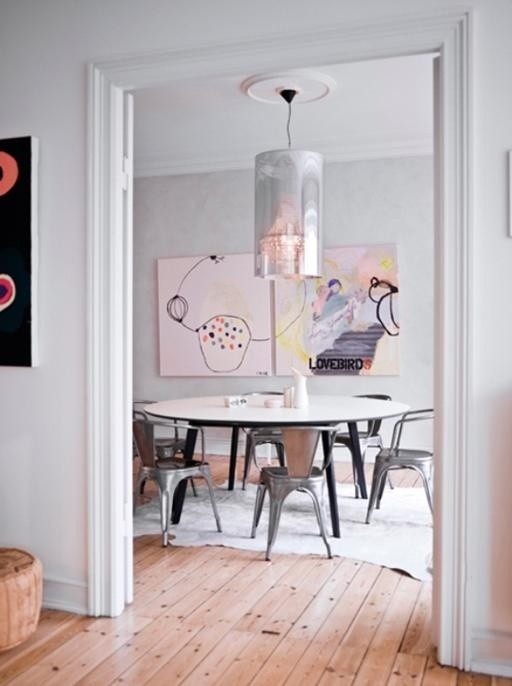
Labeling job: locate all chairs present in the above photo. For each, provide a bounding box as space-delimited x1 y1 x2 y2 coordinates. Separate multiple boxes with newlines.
133 419 222 546
365 408 436 524
246 426 340 561
132 399 197 496
240 390 290 490
242 392 286 492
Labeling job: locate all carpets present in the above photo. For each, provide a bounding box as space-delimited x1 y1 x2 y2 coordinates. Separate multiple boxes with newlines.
134 478 434 582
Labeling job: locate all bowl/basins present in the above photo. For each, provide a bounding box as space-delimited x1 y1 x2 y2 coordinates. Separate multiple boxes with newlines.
263 396 285 409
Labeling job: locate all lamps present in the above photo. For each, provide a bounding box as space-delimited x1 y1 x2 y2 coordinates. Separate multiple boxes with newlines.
255 89 322 278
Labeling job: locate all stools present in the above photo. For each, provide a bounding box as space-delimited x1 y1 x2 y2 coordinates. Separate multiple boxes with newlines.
0 546 42 652
